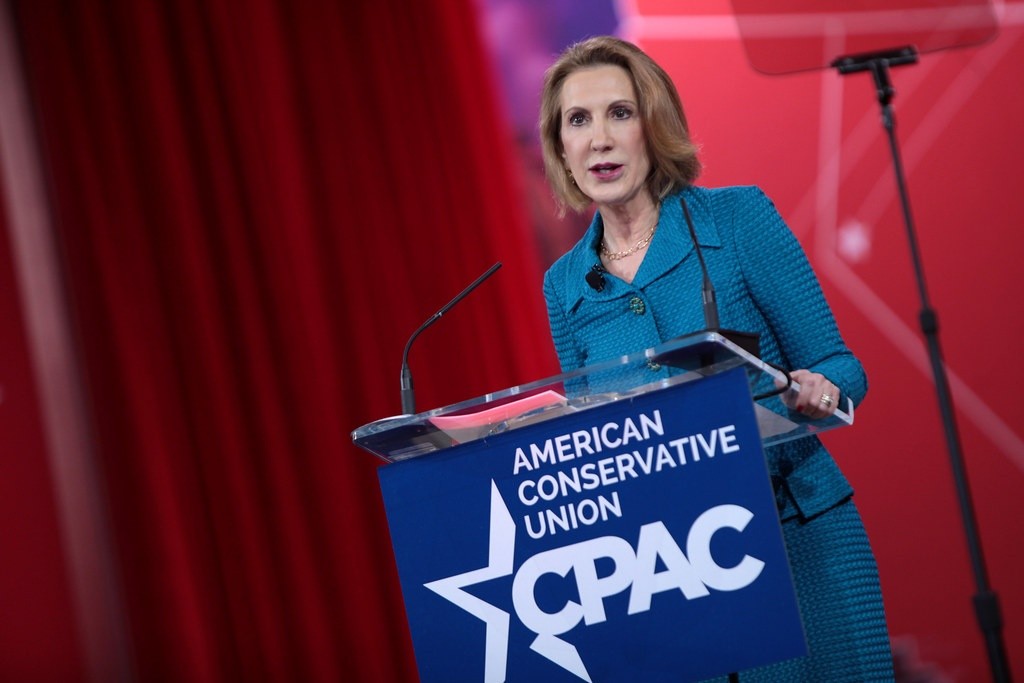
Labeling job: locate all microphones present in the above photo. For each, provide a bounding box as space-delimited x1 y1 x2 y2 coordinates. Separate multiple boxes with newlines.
645 196 761 376
350 261 502 462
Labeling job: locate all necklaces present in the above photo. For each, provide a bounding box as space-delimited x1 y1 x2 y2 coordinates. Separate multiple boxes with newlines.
600 201 661 260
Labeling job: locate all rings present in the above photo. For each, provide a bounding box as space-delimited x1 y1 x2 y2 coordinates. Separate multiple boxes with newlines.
820 394 833 408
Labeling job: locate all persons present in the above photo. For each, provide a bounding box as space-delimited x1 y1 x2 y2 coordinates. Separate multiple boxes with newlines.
538 37 895 683
